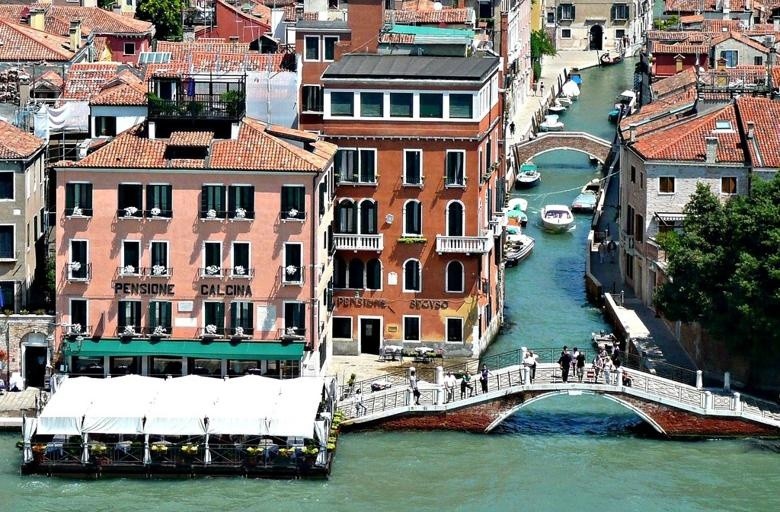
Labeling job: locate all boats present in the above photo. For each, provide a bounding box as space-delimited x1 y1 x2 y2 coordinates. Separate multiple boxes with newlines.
500 198 535 267
601 49 626 66
515 160 541 189
539 204 574 235
541 71 582 131
573 177 599 213
594 329 617 350
609 90 635 122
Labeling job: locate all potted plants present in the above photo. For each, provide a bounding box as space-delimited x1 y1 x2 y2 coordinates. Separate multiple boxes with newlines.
188 102 203 117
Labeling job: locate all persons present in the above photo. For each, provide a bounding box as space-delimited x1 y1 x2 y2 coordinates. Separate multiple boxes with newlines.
532 80 538 97
480 363 493 394
409 371 421 405
509 122 515 137
607 239 616 263
522 333 633 387
540 82 545 97
505 236 512 248
622 34 630 49
461 371 474 399
598 241 606 263
321 401 330 412
355 389 366 416
445 370 457 402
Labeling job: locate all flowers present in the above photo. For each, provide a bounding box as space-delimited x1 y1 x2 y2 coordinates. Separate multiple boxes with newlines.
80 410 342 457
